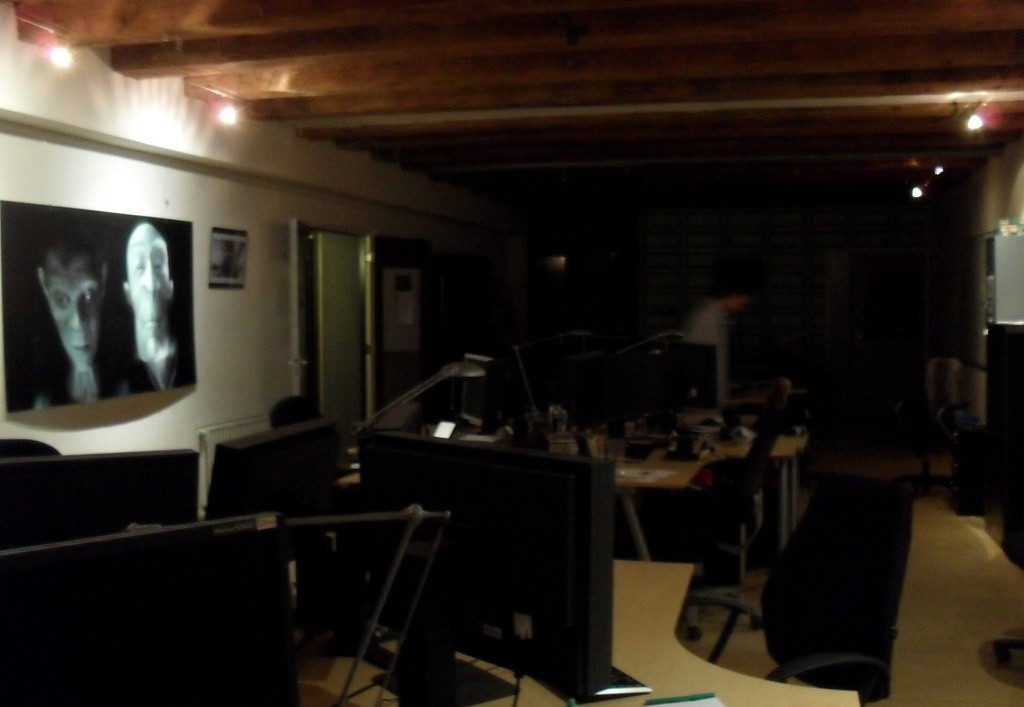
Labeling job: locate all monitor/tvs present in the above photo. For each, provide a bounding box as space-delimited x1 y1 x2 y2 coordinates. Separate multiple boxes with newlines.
0 450 199 666
202 416 342 618
359 429 616 707
461 331 717 436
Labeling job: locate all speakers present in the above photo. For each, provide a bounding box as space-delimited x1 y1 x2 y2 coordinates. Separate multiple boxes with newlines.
949 429 986 516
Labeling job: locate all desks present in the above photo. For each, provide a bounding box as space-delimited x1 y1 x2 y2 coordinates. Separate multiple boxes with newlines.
281 561 860 707
891 490 1023 707
598 428 807 572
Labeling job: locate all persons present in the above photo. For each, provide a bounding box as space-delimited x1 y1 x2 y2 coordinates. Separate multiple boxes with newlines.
681 267 753 429
32 220 196 413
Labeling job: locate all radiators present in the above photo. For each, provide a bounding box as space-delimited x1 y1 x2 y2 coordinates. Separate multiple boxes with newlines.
196 411 274 510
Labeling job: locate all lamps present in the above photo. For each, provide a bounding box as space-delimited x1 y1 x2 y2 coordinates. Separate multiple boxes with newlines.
354 361 487 439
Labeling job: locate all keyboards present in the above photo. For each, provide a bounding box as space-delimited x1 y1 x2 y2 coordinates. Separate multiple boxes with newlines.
558 664 650 697
